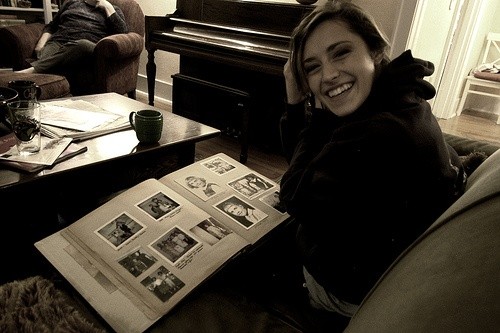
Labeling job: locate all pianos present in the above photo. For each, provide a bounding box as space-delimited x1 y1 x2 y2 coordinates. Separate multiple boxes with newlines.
145 0 318 156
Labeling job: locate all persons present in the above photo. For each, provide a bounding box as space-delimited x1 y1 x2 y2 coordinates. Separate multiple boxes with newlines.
114 222 132 238
233 175 269 196
209 0 468 333
185 176 222 198
152 272 179 295
132 252 155 272
222 202 266 224
157 232 194 257
204 225 226 239
148 198 171 214
13 0 128 90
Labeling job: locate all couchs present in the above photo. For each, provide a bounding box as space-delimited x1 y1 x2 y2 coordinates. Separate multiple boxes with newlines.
0 130 500 333
0 0 145 104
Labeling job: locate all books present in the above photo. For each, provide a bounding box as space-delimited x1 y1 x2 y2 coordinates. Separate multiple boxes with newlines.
34 151 293 333
0 142 88 174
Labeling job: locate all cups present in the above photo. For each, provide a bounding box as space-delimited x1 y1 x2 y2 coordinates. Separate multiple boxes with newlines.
8 80 42 101
129 110 163 146
7 100 41 156
0 86 20 135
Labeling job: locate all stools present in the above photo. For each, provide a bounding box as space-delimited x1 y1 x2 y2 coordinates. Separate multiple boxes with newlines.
0 70 73 100
173 67 286 163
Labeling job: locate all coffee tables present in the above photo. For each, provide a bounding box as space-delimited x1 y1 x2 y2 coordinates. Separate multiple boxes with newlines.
0 93 221 285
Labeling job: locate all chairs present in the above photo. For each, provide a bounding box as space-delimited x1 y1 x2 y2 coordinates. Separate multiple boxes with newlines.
456 35 500 124
